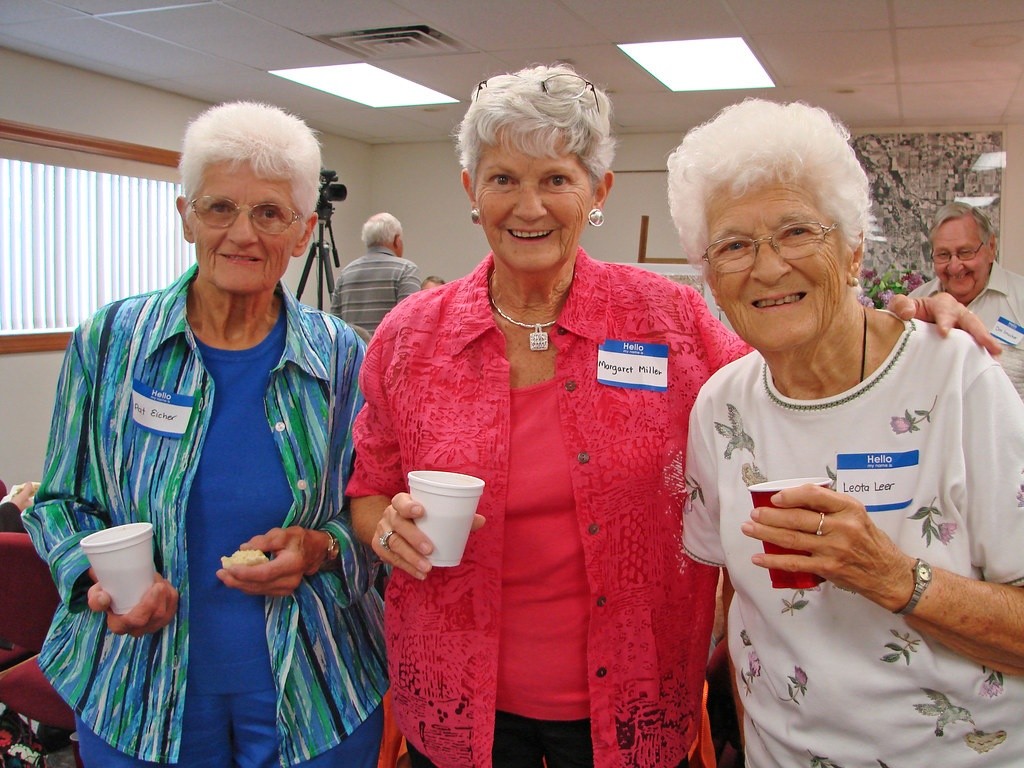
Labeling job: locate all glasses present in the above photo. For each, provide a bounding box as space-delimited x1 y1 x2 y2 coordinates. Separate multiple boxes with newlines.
704 218 845 275
472 73 604 115
186 194 303 232
926 243 990 262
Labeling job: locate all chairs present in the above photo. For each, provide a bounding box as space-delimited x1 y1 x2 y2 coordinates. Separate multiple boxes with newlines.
0 532 69 664
0 652 88 747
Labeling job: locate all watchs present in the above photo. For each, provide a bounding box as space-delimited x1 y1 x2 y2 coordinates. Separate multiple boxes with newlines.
325 530 339 561
893 558 932 616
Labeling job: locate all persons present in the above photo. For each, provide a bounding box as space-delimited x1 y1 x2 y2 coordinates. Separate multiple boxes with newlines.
0 482 34 534
664 100 1024 768
329 213 422 336
422 276 445 290
20 102 388 768
906 202 1024 404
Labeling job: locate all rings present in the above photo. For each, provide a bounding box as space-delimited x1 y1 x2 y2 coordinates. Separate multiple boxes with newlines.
380 531 396 549
816 513 824 535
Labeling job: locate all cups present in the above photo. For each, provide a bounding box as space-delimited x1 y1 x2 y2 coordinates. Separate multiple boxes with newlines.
748 479 832 589
408 470 485 567
80 522 153 615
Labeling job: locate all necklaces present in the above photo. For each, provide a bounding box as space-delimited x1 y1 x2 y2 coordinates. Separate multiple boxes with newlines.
860 310 867 383
344 65 1004 768
489 269 555 351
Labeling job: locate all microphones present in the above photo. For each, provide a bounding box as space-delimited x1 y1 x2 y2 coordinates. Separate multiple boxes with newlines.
319 170 336 177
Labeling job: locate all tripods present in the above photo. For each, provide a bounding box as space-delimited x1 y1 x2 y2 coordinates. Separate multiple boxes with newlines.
295 202 340 312
